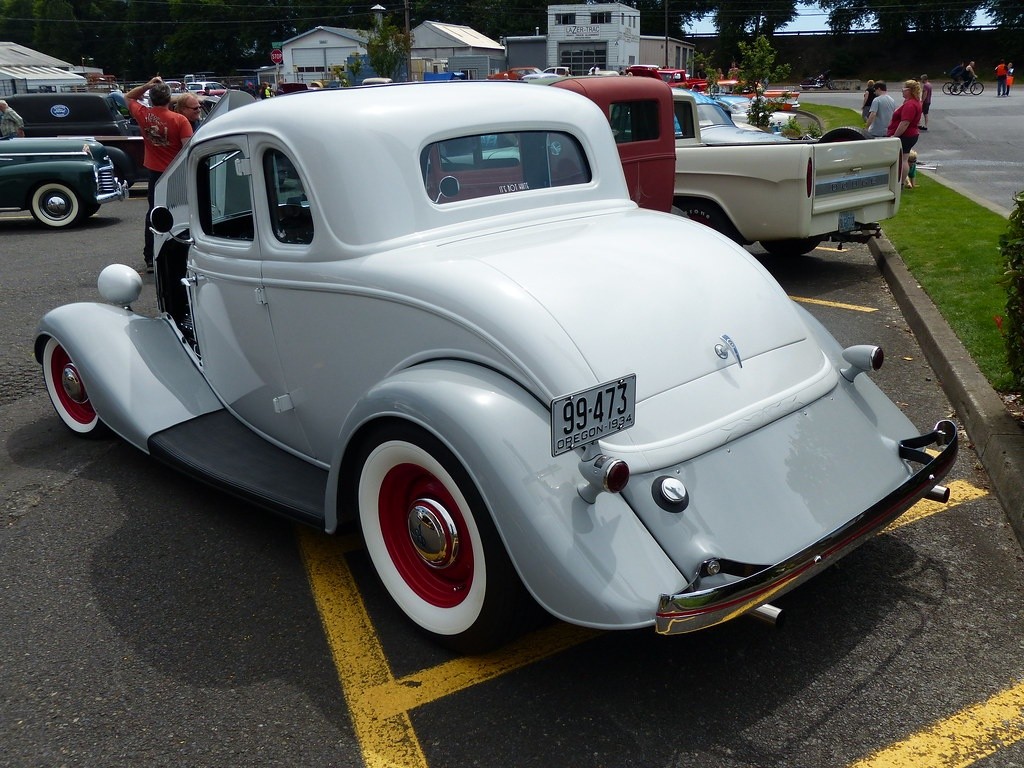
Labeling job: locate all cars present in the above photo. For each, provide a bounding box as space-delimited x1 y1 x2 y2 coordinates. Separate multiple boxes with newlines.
486 67 619 83
32 82 963 657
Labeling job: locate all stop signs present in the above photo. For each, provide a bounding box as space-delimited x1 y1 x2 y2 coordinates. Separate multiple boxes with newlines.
270 50 283 63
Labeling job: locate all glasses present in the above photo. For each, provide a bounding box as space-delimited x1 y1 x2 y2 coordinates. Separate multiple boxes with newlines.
186 105 203 111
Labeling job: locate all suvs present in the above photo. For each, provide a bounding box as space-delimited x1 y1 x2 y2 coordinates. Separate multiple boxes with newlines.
0 89 162 231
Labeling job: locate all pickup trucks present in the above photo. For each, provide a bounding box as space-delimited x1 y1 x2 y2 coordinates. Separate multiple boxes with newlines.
657 68 710 92
440 89 902 263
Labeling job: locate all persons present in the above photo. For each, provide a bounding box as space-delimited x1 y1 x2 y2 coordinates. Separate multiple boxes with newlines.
951 61 978 93
995 59 1013 97
698 62 738 92
564 63 633 77
0 100 24 138
862 74 932 188
248 80 285 100
124 76 210 274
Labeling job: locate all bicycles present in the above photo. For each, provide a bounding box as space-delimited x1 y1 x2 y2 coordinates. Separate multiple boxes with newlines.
942 76 984 96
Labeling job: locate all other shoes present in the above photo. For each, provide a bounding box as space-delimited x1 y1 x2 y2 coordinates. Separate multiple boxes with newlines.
145 260 154 272
901 183 904 190
918 125 928 130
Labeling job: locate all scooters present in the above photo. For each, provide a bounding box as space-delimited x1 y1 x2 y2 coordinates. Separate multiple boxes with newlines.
800 70 832 90
